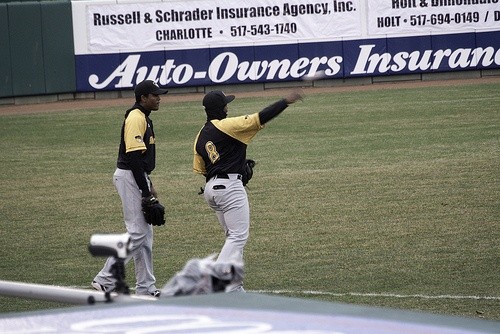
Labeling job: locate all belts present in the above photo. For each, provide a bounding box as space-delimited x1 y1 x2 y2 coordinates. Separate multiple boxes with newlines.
214 175 241 180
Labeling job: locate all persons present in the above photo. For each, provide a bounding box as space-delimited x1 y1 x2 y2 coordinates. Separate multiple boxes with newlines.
191 88 306 292
91 81 167 295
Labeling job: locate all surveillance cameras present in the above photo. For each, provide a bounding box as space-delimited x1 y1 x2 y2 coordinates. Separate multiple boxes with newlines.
90 232 134 260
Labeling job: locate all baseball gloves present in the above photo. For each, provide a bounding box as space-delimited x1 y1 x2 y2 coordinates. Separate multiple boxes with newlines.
246 159 255 184
141 197 166 226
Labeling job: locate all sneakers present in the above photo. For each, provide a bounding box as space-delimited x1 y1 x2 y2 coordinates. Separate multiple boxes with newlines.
150 289 161 298
91 281 116 292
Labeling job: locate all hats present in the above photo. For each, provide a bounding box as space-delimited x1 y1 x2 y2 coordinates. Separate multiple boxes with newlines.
134 80 168 96
203 90 235 109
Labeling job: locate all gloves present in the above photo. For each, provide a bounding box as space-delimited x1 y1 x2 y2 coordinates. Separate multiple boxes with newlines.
142 197 165 226
242 159 255 186
